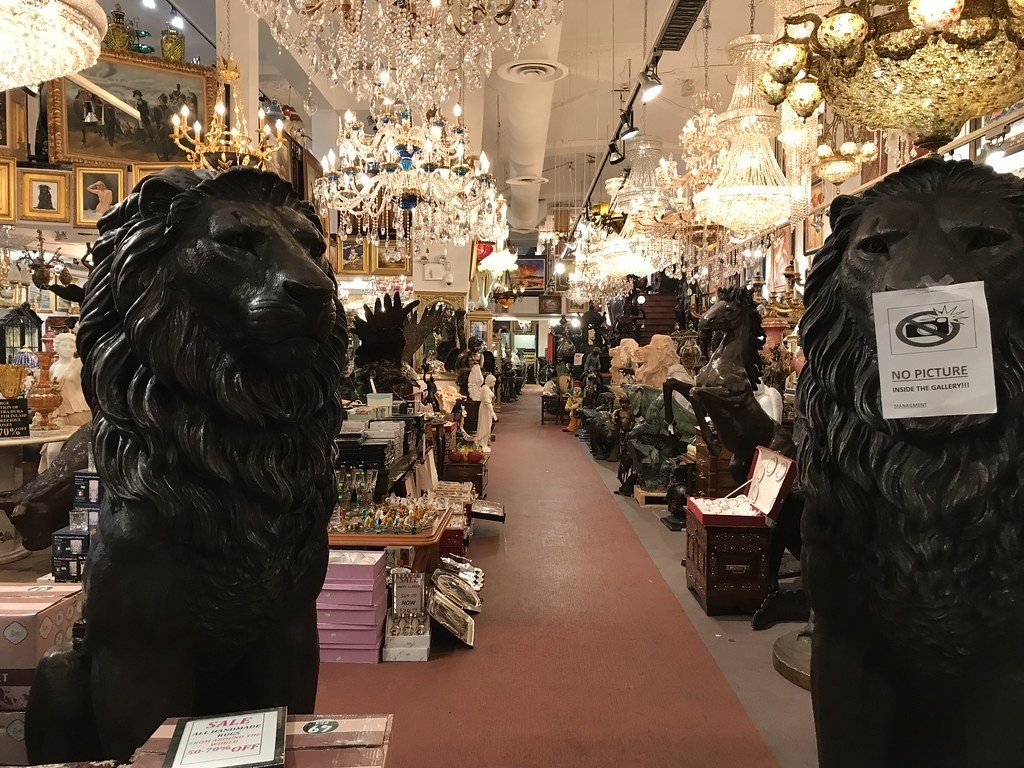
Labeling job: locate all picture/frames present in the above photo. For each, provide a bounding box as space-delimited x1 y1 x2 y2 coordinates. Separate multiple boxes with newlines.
55 275 80 312
369 240 414 277
539 295 562 314
898 136 954 167
0 155 17 222
259 112 294 187
0 88 29 162
329 237 338 275
337 235 370 275
969 99 1024 165
26 276 54 314
742 240 766 286
803 178 825 257
132 161 200 187
45 47 217 171
72 162 128 230
566 297 588 313
17 169 71 223
301 148 330 240
771 223 795 293
337 170 412 239
504 255 549 297
553 256 575 294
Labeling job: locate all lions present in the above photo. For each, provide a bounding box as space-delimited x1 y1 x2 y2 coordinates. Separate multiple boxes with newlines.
23 166 347 764
792 155 1023 768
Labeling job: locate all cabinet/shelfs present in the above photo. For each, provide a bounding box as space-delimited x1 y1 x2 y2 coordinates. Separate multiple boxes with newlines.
694 426 743 500
468 310 493 350
680 506 773 618
445 455 490 500
492 337 502 413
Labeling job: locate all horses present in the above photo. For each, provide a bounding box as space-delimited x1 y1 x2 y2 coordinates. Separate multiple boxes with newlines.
663 285 798 490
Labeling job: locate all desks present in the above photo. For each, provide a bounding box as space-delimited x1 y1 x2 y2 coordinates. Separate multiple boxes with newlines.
386 445 422 490
0 425 81 564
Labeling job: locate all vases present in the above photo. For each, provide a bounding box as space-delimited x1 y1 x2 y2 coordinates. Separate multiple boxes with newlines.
26 351 63 431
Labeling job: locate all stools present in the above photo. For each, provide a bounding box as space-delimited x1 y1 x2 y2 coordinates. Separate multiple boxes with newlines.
540 395 560 426
561 394 571 426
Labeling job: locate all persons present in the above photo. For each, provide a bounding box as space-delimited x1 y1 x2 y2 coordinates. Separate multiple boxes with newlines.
584 347 602 372
477 375 497 453
613 302 645 339
455 336 484 393
612 397 635 436
562 387 583 433
37 333 92 475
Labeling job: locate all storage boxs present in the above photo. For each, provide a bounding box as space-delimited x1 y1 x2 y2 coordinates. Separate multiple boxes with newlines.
315 393 506 664
0 442 100 765
689 445 798 526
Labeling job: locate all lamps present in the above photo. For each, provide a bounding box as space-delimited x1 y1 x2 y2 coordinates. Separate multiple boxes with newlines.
0 0 1024 306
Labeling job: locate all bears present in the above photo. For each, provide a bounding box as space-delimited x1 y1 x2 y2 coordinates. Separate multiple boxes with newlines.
588 411 620 462
